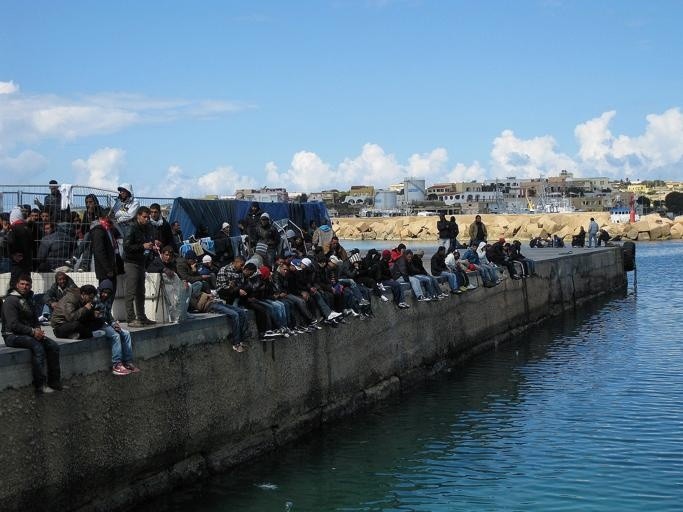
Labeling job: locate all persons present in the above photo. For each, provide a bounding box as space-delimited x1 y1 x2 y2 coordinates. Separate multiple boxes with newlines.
178 202 373 352
432 213 534 294
1 179 184 393
540 233 552 247
576 216 611 248
362 244 448 309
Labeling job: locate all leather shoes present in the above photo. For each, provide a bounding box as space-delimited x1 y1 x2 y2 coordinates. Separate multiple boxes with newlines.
143 315 156 325
128 320 145 327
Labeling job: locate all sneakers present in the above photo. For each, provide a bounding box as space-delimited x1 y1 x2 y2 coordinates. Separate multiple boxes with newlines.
259 299 373 345
65 258 77 267
241 341 249 351
50 382 68 392
35 384 55 395
398 301 411 309
381 296 389 302
78 267 85 272
418 291 449 302
453 284 476 295
232 343 244 353
485 278 503 288
512 273 530 280
378 283 385 291
112 360 130 376
124 360 141 374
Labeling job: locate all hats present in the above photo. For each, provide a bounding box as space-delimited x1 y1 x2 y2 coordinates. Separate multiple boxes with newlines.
202 254 212 264
260 212 270 221
290 258 302 271
185 249 198 260
302 258 312 267
